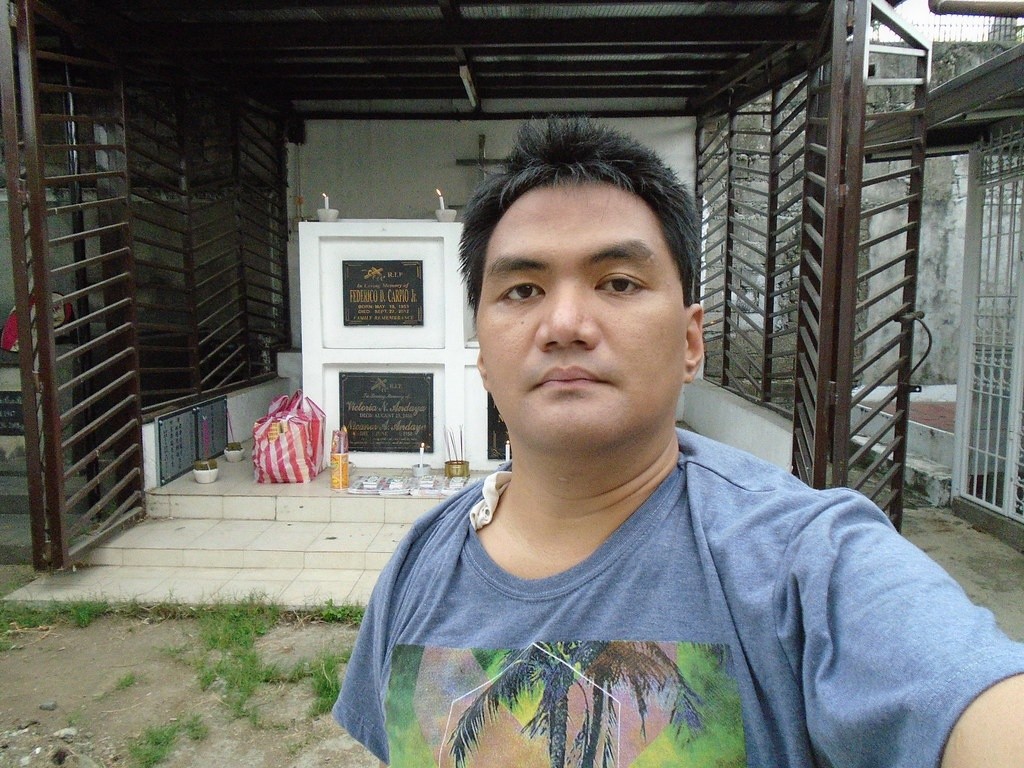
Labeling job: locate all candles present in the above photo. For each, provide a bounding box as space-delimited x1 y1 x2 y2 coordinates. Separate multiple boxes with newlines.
419 443 424 468
323 193 330 210
437 189 445 210
505 441 510 463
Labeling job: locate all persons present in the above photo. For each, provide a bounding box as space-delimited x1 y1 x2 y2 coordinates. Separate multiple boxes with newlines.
332 112 1024 768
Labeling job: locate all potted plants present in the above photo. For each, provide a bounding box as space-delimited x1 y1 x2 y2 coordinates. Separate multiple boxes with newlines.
194 460 218 483
224 442 244 462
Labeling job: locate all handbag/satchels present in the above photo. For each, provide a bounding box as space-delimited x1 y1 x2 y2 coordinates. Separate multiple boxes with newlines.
252 389 327 484
